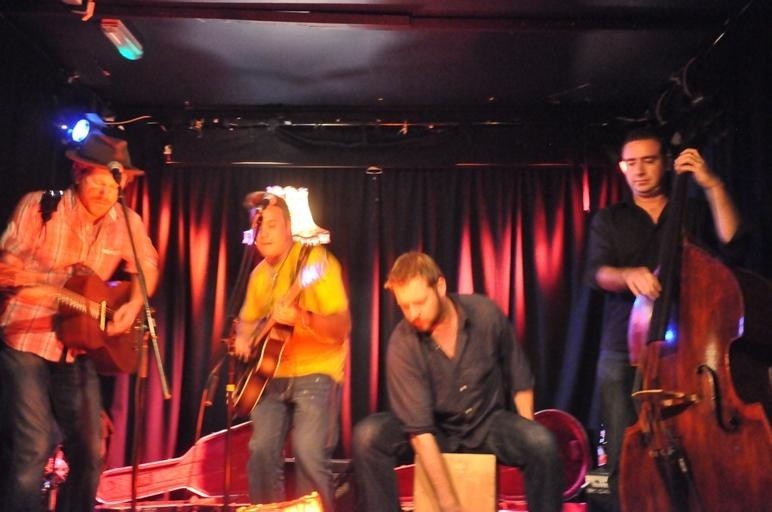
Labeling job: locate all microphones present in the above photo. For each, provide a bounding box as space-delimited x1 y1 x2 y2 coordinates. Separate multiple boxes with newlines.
108 160 123 186
255 193 278 213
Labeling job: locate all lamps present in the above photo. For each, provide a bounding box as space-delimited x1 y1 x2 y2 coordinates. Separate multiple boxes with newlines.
263 184 331 247
99 19 144 62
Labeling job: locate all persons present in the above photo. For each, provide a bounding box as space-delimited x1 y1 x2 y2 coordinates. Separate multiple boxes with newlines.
222 189 355 511
347 248 567 512
0 133 164 512
579 127 744 512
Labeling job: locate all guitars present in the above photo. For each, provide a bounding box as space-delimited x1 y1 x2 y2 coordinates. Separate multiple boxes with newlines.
226 263 327 418
0 251 146 375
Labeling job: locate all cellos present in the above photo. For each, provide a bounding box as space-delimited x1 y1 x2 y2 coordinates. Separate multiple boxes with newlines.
617 94 772 512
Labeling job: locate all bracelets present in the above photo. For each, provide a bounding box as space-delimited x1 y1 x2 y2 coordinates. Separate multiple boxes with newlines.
701 179 722 191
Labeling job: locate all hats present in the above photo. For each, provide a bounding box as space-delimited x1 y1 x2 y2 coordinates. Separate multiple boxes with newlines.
66 134 145 175
